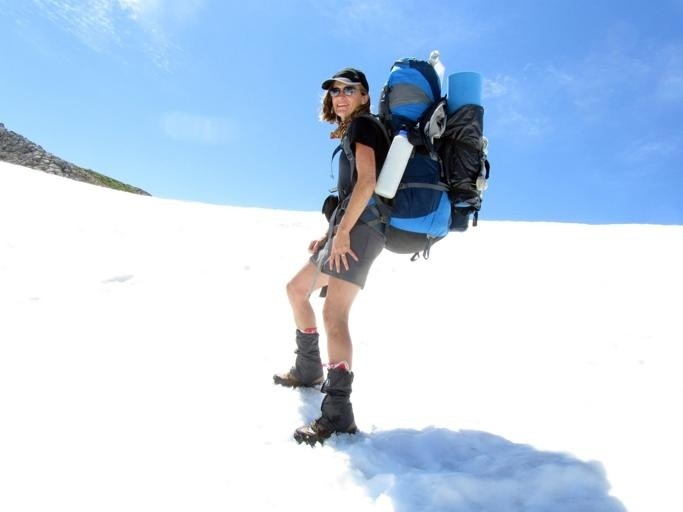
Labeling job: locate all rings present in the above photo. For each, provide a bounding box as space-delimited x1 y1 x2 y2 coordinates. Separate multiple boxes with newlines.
341 254 345 257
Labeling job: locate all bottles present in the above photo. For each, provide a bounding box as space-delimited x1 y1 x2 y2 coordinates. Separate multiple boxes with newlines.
373 130 415 198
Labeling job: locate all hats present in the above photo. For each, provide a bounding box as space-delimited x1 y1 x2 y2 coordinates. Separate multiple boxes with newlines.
322 68 369 92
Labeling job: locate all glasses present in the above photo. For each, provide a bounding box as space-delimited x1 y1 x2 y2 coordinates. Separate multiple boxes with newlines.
328 85 366 98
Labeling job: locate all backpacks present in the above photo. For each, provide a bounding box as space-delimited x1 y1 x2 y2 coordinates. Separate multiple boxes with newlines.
343 58 452 252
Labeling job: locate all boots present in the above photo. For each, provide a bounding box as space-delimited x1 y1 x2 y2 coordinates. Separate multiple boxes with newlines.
294 362 357 444
272 326 323 387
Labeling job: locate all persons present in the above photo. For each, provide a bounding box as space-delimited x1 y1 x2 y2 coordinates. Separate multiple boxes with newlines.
274 69 392 445
